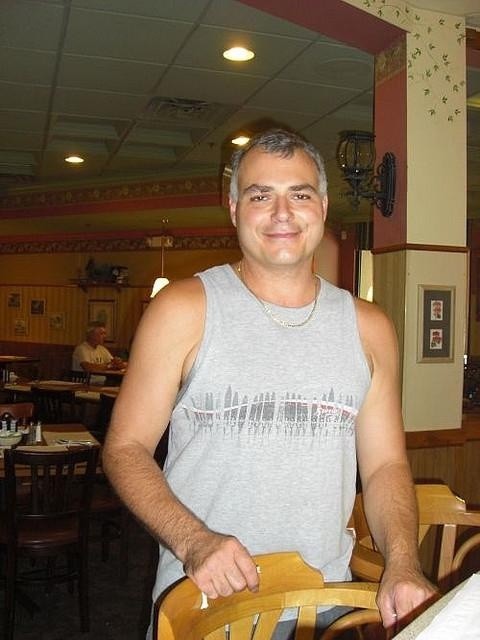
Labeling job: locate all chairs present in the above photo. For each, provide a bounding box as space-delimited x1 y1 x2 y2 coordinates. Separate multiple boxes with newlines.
149 550 431 640
338 481 478 592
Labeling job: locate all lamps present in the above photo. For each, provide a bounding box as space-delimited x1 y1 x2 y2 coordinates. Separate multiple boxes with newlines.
148 216 171 299
334 130 394 219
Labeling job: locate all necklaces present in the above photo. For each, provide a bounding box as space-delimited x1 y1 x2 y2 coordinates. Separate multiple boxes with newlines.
234 252 322 329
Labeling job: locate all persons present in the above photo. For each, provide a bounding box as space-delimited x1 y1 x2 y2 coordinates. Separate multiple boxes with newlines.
73 315 129 390
101 126 446 640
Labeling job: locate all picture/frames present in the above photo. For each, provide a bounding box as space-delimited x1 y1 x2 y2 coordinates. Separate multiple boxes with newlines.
417 282 456 362
47 311 66 331
87 299 116 343
6 292 22 310
30 298 46 315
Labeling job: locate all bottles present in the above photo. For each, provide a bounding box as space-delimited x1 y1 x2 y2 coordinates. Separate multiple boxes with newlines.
0 416 42 446
